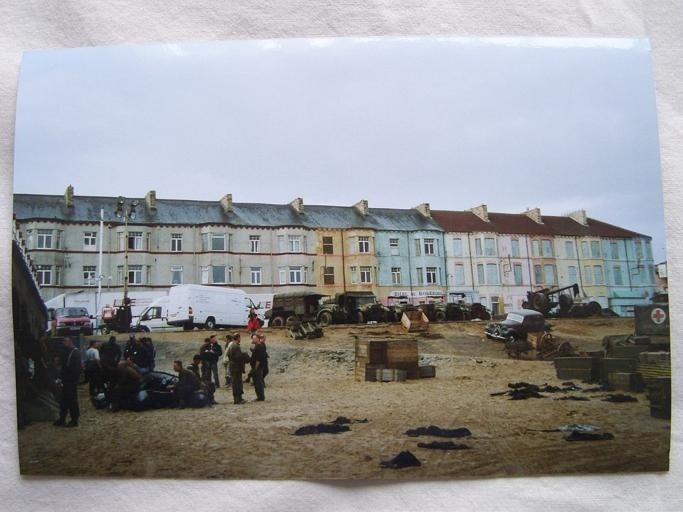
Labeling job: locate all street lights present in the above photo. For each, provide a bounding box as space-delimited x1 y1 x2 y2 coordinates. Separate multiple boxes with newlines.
114 193 139 305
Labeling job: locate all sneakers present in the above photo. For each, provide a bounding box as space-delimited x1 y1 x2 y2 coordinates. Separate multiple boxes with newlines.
235 394 265 405
52 418 80 429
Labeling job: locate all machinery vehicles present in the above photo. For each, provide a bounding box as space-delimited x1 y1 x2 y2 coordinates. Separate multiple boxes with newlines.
522 284 601 319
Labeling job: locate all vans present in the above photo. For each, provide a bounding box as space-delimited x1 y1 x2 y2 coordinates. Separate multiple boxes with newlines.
133 295 184 334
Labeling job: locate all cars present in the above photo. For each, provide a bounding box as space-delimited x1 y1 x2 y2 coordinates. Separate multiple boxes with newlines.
482 308 549 342
19 332 92 385
50 305 94 334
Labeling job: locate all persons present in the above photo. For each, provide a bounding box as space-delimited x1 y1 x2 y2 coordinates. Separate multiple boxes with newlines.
83 331 269 403
31 333 56 396
246 308 263 338
50 333 82 428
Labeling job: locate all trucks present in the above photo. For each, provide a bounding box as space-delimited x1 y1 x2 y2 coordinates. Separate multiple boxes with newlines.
264 290 490 326
166 282 265 334
